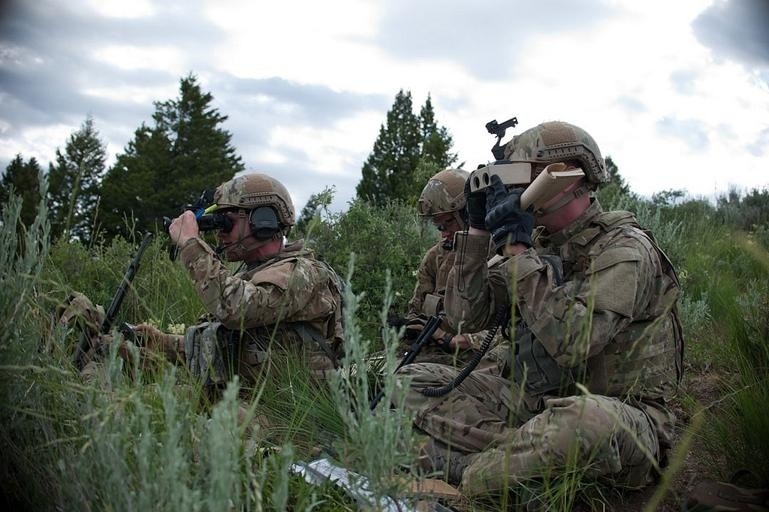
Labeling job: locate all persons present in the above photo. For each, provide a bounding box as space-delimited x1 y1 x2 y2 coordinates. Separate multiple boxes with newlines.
349 166 516 399
382 118 686 511
72 170 352 459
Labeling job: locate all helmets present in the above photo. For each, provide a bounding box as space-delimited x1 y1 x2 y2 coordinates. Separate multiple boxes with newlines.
213 172 295 227
507 120 612 186
419 168 472 217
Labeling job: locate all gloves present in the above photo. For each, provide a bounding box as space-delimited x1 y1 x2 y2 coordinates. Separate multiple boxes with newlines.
464 164 534 256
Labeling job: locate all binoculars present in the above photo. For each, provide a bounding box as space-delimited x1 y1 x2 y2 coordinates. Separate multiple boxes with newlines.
162 213 234 234
470 163 532 194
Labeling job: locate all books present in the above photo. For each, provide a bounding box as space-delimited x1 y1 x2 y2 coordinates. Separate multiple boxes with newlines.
381 471 463 501
520 162 586 213
